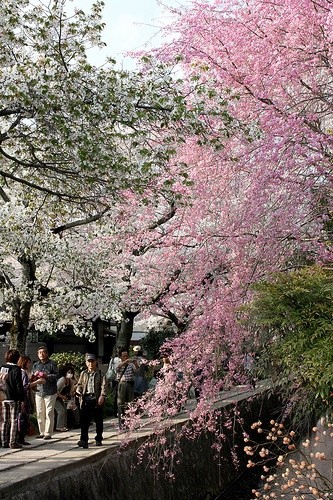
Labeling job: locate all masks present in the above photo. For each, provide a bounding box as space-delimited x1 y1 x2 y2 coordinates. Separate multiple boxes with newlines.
66 372 73 379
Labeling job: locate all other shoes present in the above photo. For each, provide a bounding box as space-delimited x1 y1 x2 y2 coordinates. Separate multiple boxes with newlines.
42 435 52 440
10 444 23 448
36 435 44 438
78 440 88 449
21 442 30 445
59 429 67 432
96 440 102 446
63 428 70 431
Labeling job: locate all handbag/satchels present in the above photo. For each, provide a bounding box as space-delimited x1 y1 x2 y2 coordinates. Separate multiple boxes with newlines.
112 383 117 398
106 357 116 380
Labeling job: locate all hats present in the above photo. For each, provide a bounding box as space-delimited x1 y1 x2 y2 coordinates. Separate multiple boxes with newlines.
85 353 96 361
132 346 141 351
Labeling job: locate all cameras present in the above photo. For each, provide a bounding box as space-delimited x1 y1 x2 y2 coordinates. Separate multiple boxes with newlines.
129 359 135 363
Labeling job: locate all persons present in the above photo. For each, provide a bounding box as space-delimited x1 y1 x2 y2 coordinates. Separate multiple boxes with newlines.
74 353 107 449
105 345 258 431
0 350 24 448
53 363 80 431
29 346 59 439
15 356 46 446
